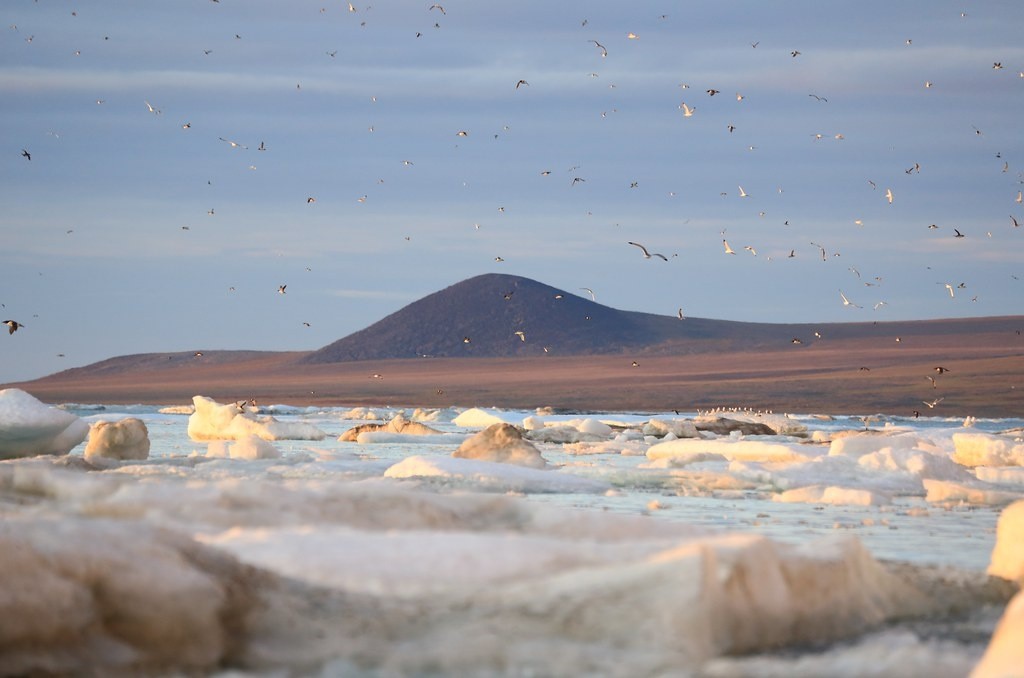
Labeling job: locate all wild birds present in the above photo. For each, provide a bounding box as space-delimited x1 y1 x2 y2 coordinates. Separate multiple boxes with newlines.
0 0 1024 432
